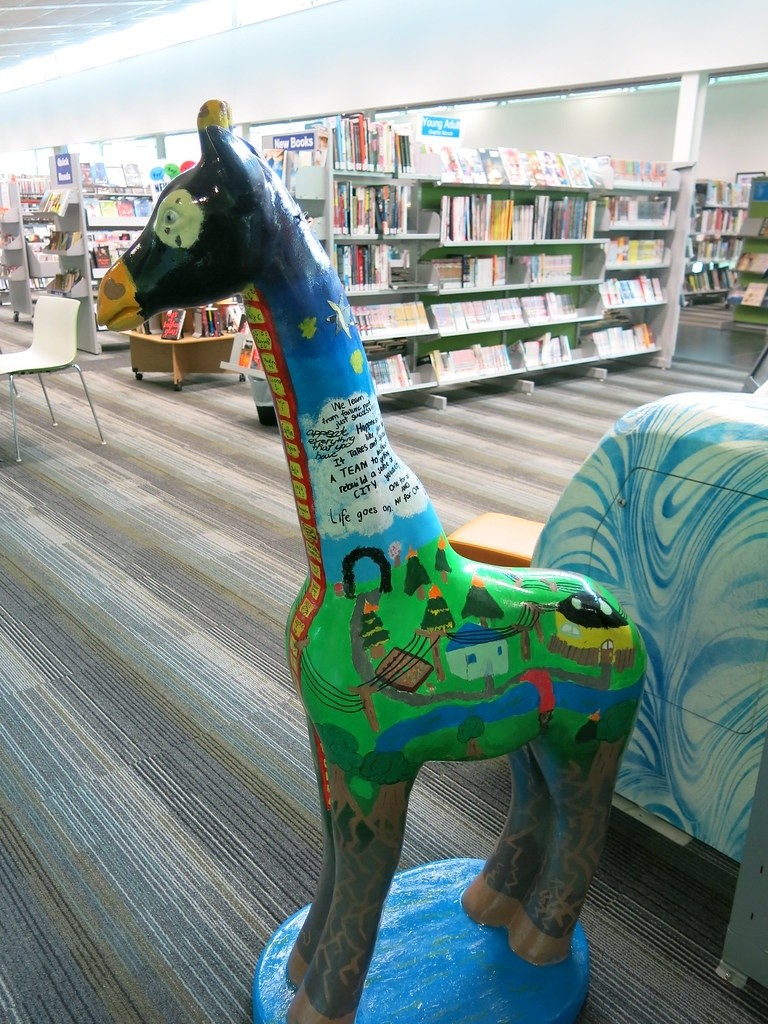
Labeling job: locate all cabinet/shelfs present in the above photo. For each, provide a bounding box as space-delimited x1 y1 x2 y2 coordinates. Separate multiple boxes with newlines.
121 293 247 391
31 152 204 356
681 177 752 309
731 174 768 334
0 174 61 325
220 112 697 411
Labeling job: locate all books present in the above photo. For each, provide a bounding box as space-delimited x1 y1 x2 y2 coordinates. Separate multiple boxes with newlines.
0 174 50 292
415 145 672 385
332 113 415 395
727 281 768 307
238 313 266 371
55 265 81 294
752 180 768 201
92 278 110 332
49 230 82 252
133 293 246 340
83 195 154 225
738 217 768 237
262 116 331 192
42 190 66 213
735 252 768 273
87 228 145 268
79 162 152 194
684 178 751 293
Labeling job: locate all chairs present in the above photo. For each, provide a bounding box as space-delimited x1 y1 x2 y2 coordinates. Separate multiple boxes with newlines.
0 296 107 462
